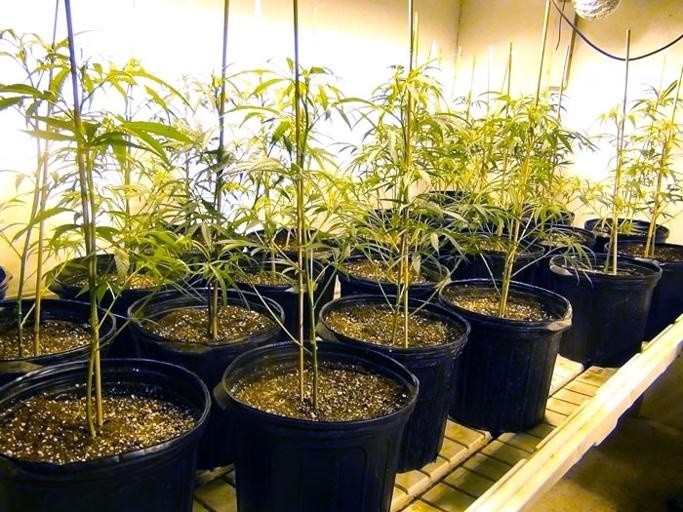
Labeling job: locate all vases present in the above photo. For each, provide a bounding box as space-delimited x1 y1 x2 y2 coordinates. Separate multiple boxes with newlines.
0 264 12 291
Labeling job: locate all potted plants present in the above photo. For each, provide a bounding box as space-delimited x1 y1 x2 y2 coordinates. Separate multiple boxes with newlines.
319 65 471 474
583 80 672 240
43 59 191 315
0 27 115 379
549 73 662 369
439 95 573 433
603 79 683 341
215 61 421 512
0 43 212 512
